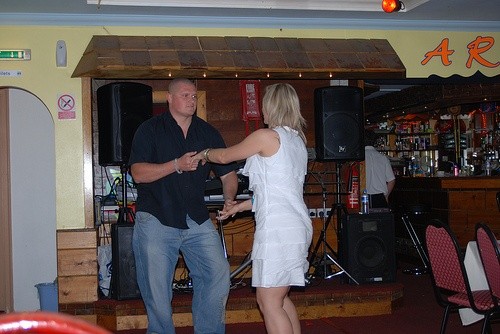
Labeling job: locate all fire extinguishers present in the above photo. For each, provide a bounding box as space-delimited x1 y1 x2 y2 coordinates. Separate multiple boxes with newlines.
345 163 361 209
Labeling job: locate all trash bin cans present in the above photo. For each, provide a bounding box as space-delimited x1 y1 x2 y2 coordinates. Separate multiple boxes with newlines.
34 283 59 313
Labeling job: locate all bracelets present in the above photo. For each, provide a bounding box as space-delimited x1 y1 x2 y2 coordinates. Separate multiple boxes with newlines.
174 158 182 175
204 148 209 163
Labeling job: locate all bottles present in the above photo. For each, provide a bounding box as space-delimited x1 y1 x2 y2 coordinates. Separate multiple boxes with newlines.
402 165 406 176
453 164 458 178
484 161 491 177
361 189 370 214
467 164 471 177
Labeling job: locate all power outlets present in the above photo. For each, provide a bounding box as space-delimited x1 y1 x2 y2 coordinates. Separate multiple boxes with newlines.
317 208 331 217
308 209 316 218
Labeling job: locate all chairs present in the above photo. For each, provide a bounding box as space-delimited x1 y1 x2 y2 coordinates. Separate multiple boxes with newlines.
424 218 500 334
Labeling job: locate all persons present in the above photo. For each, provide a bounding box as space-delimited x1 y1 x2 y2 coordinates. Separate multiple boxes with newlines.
363 129 395 208
129 78 240 334
191 83 314 334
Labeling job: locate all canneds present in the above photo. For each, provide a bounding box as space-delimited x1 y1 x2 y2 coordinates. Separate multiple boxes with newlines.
453 165 459 176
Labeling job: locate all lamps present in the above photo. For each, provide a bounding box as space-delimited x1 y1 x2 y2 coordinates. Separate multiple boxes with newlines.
382 0 406 13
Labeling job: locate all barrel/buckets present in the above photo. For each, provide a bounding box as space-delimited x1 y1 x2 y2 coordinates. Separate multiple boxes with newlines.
33 283 58 312
